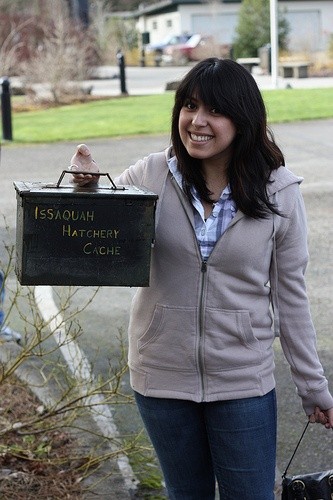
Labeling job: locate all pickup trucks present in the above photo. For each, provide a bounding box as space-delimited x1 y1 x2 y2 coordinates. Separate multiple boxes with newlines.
165 34 231 66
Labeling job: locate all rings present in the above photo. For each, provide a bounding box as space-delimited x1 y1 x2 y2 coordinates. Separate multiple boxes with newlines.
68 164 78 171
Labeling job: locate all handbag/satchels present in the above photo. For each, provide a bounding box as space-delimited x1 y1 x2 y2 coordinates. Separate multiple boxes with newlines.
282 422 333 500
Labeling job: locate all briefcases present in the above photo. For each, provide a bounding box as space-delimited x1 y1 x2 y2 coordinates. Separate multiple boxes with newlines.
12 171 160 288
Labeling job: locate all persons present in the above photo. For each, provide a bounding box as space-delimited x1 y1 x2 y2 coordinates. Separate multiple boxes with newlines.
67 54 333 500
